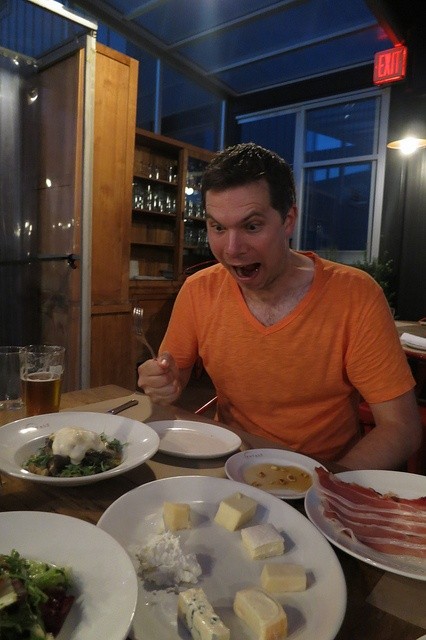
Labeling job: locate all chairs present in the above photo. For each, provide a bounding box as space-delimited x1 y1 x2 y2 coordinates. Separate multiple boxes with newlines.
194 395 425 474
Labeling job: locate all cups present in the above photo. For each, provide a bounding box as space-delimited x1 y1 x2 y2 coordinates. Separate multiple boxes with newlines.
20 343 67 419
1 343 28 413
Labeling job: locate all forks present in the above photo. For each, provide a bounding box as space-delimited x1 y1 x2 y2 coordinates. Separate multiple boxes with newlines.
129 305 164 365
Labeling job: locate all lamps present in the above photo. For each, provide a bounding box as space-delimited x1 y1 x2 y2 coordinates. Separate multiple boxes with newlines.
385 118 426 152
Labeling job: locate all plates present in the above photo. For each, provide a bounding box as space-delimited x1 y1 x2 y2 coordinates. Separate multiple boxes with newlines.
141 419 243 458
304 467 426 580
95 474 347 640
1 411 162 487
0 508 140 640
222 449 331 500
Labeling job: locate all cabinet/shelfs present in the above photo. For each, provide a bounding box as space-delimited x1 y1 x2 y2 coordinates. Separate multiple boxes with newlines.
129 128 220 393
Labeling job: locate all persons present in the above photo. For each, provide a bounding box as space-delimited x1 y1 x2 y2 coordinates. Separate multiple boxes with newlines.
137 143 422 473
